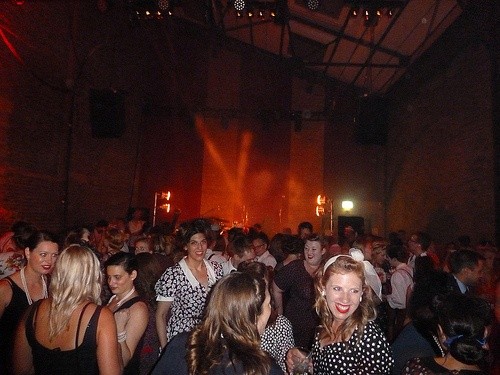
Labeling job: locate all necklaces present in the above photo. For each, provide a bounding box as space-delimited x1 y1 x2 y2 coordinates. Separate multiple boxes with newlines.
22 266 47 304
108 286 135 306
20 266 48 305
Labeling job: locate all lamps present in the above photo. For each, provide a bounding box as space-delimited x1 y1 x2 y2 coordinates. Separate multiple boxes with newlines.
314 194 335 238
152 189 170 229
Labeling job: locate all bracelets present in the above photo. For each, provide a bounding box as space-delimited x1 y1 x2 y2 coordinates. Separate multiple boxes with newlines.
118 330 126 343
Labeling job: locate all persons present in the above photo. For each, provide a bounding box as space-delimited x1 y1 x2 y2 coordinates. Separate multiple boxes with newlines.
285 248 395 375
447 249 485 310
150 271 285 375
100 228 124 307
122 252 162 375
401 295 500 375
13 245 124 375
272 233 326 355
104 251 149 372
154 227 225 349
0 230 59 375
0 210 500 373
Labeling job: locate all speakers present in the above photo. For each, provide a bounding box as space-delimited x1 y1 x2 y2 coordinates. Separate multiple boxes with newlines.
352 94 389 145
338 216 365 244
89 87 125 138
